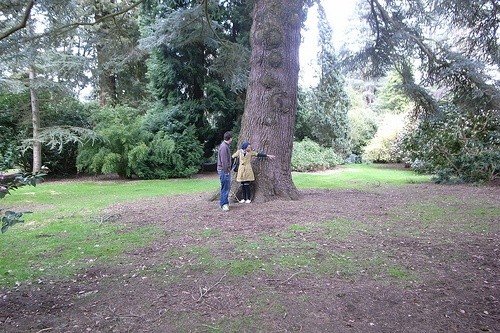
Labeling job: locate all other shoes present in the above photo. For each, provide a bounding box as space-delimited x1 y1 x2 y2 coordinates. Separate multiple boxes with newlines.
239 200 245 204
222 204 229 211
245 200 251 204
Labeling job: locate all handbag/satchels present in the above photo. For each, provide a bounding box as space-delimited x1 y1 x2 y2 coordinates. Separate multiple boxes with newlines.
233 158 239 172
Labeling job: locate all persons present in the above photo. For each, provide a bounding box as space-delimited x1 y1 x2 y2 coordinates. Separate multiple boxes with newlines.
218 131 234 212
232 142 275 203
